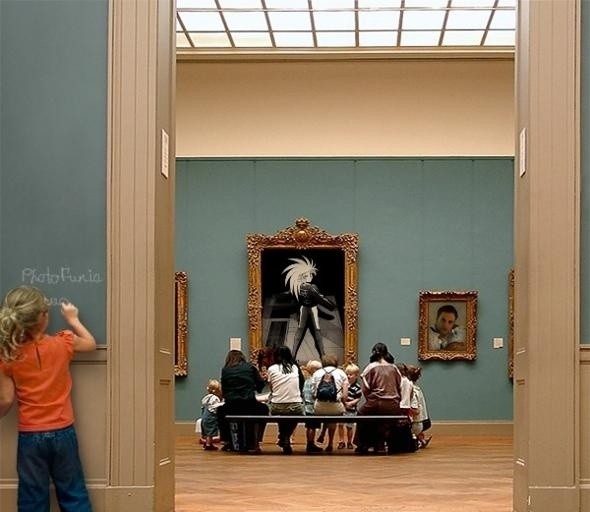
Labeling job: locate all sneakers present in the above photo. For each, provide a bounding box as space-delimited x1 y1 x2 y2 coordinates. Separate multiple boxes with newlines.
305 436 368 454
277 434 292 453
417 433 433 448
199 437 261 454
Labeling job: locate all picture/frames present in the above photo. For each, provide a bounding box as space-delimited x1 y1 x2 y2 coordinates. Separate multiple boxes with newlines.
173 270 189 377
245 216 360 383
417 289 478 363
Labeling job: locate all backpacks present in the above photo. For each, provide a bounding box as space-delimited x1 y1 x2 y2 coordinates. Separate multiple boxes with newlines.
316 368 343 402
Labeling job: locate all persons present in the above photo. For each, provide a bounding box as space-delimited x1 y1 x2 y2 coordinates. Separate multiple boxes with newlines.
394 362 423 450
351 342 418 455
215 349 270 453
336 362 362 450
266 344 305 454
311 352 349 452
277 256 337 362
407 364 432 448
198 378 225 450
428 304 467 352
0 283 96 511
299 359 324 452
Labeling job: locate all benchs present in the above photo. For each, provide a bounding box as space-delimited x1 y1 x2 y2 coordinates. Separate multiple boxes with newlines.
224 413 408 456
